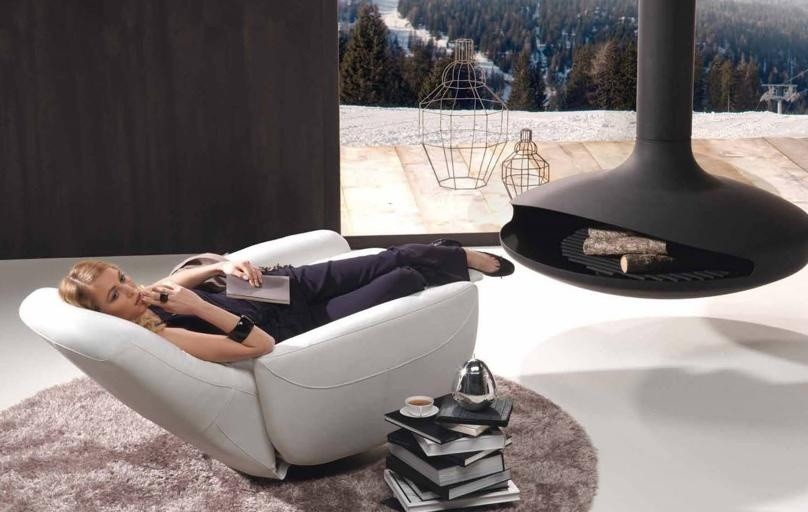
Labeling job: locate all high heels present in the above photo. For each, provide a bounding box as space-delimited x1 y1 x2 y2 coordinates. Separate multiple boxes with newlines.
468 250 514 277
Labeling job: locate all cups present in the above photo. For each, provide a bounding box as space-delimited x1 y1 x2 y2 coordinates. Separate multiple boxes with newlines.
404 395 435 416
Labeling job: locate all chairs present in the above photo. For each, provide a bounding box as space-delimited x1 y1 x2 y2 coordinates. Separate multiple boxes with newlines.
17 230 479 481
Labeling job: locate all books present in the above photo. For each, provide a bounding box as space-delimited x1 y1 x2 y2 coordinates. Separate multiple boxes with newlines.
226 275 290 307
384 395 522 512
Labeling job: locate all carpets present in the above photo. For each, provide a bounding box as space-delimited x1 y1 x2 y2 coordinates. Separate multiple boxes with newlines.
0 372 598 509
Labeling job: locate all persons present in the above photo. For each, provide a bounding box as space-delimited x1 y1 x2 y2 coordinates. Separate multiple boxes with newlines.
56 238 514 363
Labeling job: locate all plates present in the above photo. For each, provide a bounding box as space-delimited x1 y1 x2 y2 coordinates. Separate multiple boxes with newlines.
398 406 439 419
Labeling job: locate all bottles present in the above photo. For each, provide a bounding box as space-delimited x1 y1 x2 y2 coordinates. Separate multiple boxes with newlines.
450 352 498 413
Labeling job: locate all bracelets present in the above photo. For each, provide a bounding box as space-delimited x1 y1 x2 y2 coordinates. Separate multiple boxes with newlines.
228 315 255 344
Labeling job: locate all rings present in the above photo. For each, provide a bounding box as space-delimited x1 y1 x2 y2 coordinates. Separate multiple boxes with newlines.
160 293 169 302
